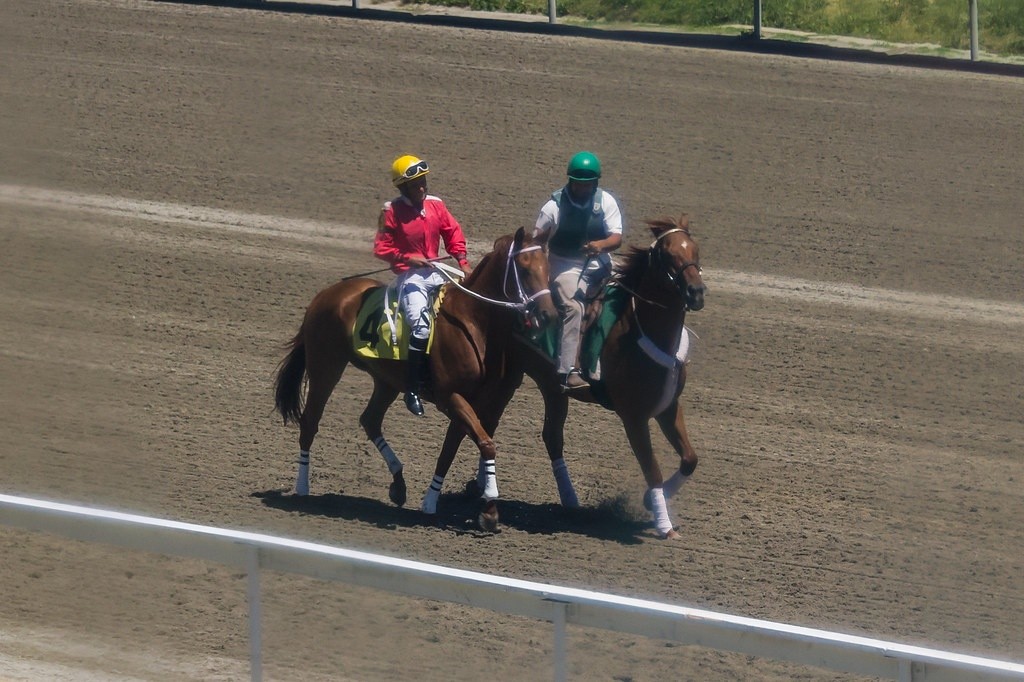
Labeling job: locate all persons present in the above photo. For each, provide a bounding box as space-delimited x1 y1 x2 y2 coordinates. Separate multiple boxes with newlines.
373 155 473 416
533 151 622 393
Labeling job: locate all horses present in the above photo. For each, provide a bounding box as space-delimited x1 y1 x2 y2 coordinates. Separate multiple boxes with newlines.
271 214 707 540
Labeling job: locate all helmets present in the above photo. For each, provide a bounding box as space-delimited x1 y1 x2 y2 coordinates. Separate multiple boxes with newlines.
567 152 601 181
391 155 429 186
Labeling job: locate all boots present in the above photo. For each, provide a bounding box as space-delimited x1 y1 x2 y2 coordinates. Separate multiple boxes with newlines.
404 348 430 416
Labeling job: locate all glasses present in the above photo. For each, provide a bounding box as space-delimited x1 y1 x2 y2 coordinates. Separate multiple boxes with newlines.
394 160 428 183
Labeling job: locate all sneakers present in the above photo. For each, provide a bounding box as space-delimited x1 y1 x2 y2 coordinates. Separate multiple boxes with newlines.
560 373 590 389
